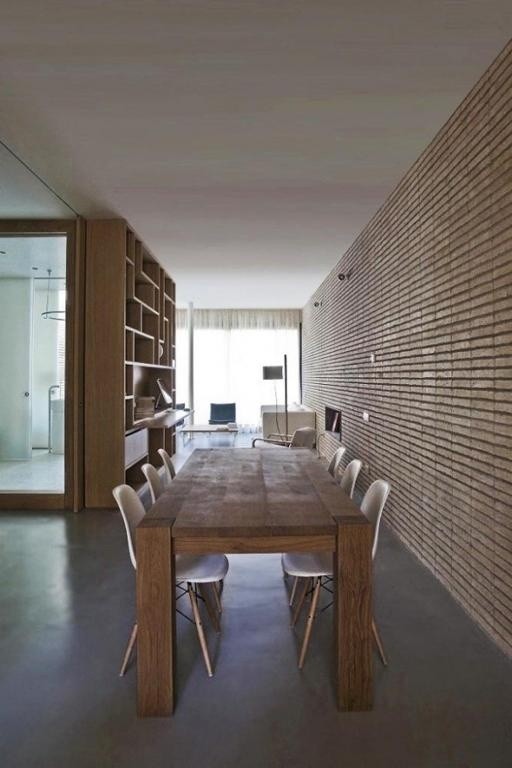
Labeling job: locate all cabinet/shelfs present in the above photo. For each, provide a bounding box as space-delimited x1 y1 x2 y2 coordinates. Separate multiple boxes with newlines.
83 218 175 509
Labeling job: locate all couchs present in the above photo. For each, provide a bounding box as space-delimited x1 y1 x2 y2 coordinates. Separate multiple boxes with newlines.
261 404 316 441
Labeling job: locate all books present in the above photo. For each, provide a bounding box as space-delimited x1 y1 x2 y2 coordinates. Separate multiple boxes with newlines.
133 395 156 418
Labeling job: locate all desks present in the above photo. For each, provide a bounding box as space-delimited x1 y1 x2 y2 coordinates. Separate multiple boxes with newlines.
135 406 195 465
135 449 373 717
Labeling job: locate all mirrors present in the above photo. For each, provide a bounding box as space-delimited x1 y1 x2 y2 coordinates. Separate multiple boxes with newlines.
0 219 76 511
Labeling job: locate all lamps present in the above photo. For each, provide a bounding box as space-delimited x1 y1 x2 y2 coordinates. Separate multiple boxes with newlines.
263 354 288 441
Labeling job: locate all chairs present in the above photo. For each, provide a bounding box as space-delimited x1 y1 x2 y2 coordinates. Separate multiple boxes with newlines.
112 446 392 679
252 427 316 450
208 403 236 435
